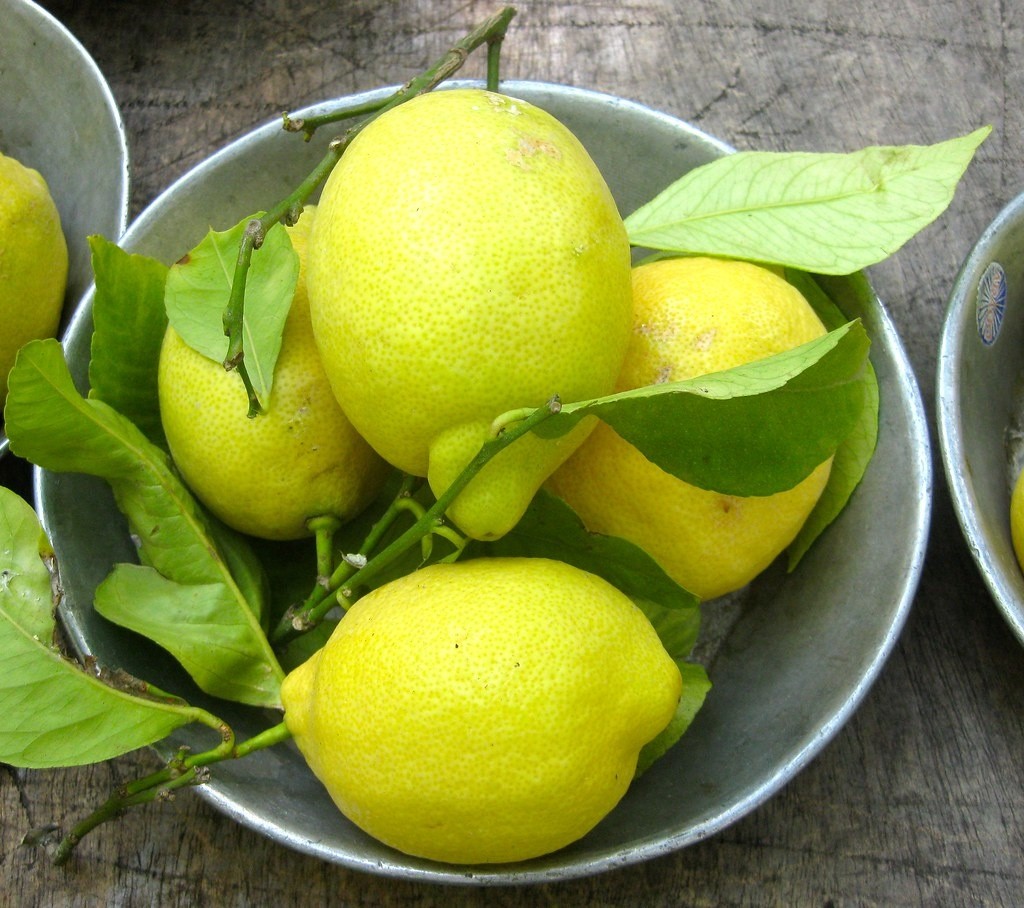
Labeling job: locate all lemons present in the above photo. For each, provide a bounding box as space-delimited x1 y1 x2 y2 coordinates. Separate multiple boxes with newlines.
158 90 837 864
0 149 70 437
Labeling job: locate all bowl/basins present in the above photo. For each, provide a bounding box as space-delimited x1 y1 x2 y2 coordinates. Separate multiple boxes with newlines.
937 190 1024 644
31 78 935 887
0 0 130 455
